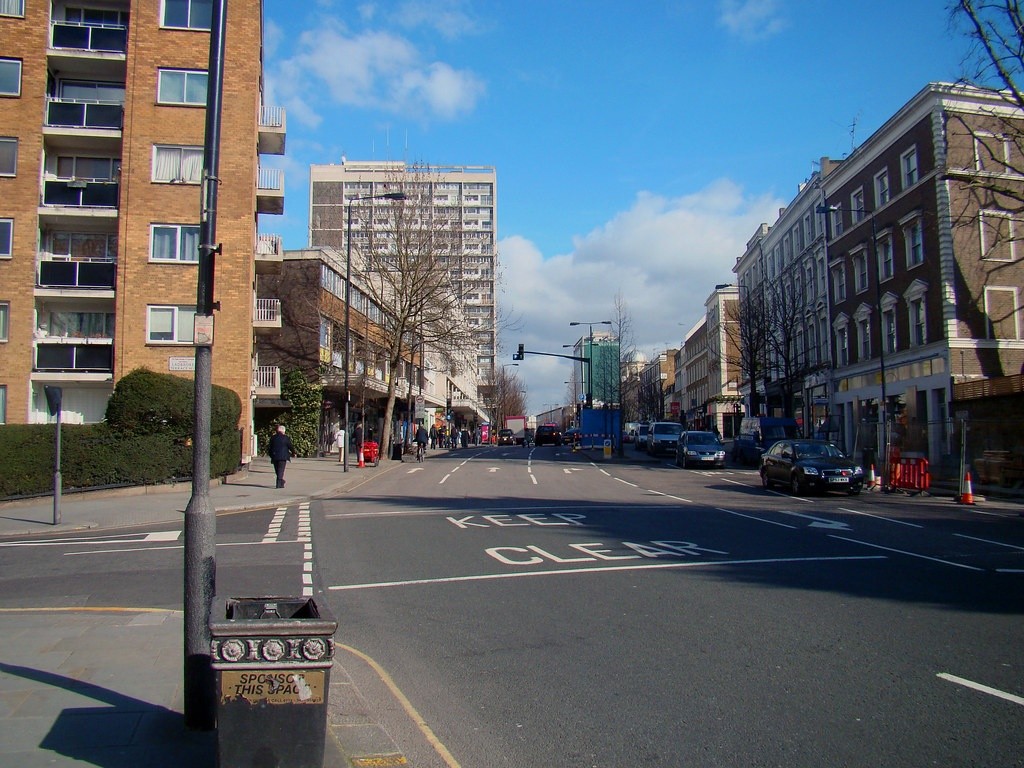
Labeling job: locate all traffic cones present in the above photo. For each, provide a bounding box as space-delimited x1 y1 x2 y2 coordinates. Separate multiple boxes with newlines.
865 464 876 491
957 472 976 505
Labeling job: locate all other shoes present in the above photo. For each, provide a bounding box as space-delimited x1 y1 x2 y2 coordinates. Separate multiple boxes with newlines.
276 486 284 488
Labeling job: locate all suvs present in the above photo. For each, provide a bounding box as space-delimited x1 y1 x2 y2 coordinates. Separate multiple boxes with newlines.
534 425 561 446
498 429 516 445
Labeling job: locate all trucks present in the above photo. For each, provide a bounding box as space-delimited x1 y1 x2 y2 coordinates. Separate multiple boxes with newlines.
625 423 640 440
504 416 526 445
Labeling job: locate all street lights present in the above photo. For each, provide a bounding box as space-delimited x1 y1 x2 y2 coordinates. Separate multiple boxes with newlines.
565 382 588 416
344 193 407 474
716 283 757 417
816 205 889 462
563 344 599 406
503 364 519 418
571 321 612 408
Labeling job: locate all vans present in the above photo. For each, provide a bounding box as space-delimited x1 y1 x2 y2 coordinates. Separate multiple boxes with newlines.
733 417 802 466
647 422 684 455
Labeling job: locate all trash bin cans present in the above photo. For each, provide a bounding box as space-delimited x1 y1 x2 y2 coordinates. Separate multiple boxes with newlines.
391 443 403 460
207 593 340 768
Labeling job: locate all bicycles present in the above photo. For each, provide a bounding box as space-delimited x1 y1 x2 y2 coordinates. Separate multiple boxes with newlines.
416 441 426 463
430 437 436 450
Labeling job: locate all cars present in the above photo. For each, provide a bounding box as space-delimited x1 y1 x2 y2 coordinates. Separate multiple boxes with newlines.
676 431 726 468
562 429 577 446
759 439 865 497
622 430 629 442
633 425 647 448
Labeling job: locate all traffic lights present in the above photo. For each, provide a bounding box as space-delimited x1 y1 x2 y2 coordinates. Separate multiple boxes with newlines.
513 344 524 360
586 393 592 404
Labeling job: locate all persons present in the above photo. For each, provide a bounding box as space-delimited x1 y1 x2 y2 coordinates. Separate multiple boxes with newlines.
367 426 373 441
460 427 481 448
635 431 638 436
700 424 706 431
429 424 439 449
687 424 692 431
334 427 345 462
437 425 446 448
351 424 362 462
413 424 428 460
269 425 295 488
525 428 530 448
712 425 721 445
450 423 462 449
